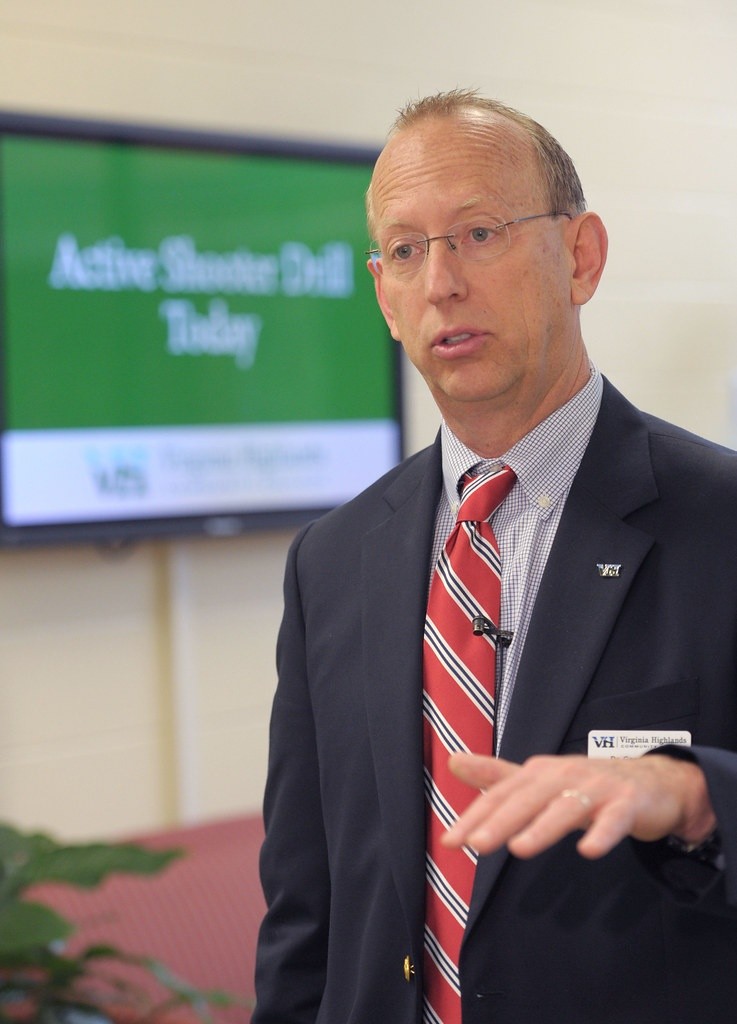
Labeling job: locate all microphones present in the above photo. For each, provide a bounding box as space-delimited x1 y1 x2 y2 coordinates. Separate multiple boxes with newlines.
473 616 485 636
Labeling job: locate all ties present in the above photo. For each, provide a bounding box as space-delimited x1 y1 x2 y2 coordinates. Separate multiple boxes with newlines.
420 463 518 1024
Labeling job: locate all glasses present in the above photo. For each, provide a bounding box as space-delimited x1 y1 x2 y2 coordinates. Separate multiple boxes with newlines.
363 210 575 279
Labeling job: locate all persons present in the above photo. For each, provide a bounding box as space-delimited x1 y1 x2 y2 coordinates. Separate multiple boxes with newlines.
254 90 737 1024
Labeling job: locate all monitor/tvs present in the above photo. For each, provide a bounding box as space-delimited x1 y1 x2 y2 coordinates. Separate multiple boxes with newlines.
0 110 407 548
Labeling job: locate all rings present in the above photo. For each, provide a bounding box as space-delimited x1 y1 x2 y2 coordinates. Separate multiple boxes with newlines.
560 789 595 815
